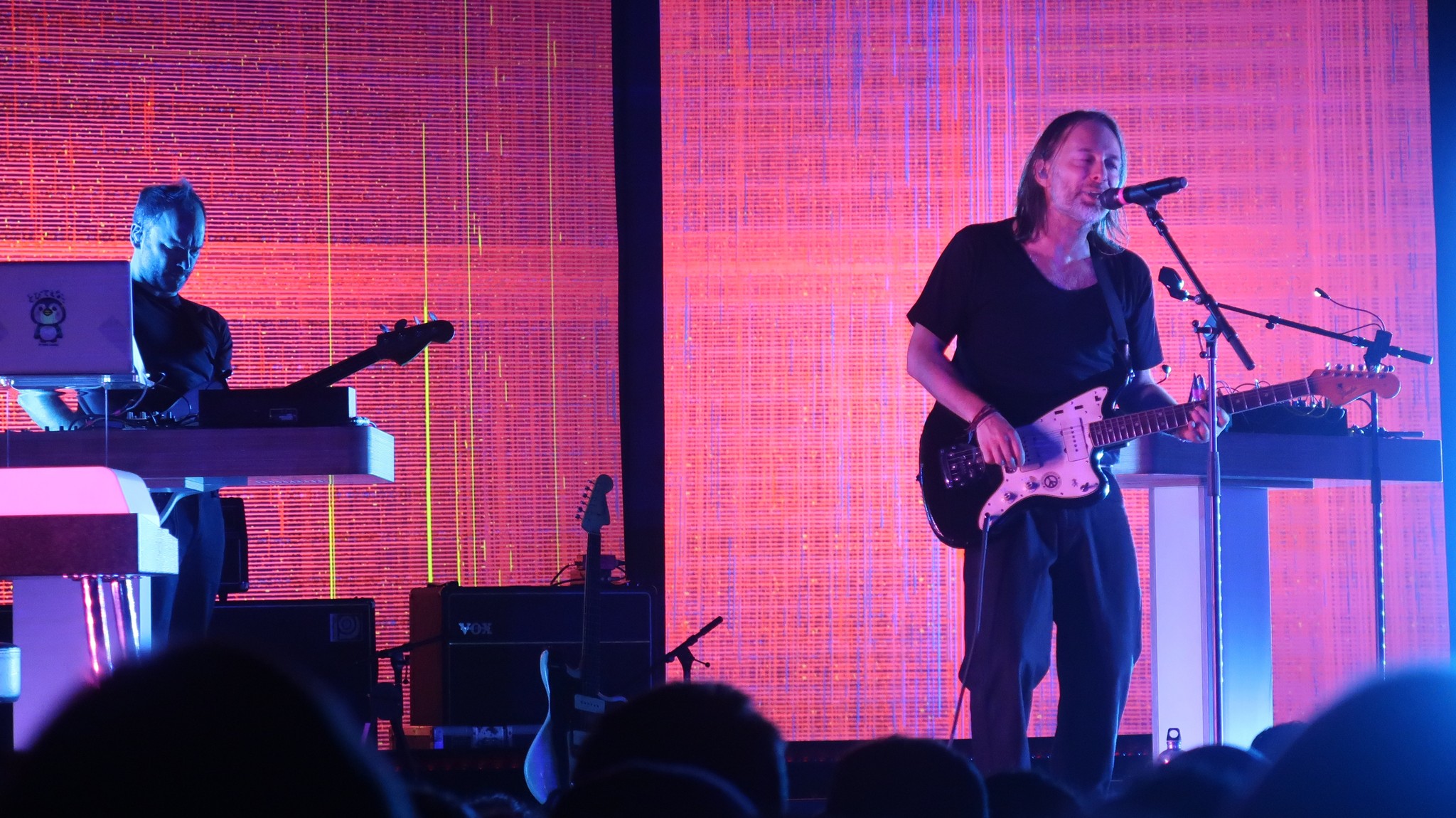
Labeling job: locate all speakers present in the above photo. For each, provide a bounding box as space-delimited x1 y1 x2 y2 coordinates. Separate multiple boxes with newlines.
405 583 659 730
204 598 376 747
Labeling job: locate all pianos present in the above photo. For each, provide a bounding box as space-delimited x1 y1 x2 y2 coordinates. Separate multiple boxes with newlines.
1103 416 1444 493
0 416 399 497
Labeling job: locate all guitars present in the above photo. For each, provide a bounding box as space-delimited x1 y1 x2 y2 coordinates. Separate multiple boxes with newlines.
85 313 457 500
524 473 631 804
915 359 1406 550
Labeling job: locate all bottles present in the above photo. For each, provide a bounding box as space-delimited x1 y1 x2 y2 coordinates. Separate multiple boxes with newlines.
1155 726 1184 766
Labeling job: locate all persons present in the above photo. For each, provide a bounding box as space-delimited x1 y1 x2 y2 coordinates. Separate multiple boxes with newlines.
16 178 233 658
904 108 1231 801
0 638 1454 818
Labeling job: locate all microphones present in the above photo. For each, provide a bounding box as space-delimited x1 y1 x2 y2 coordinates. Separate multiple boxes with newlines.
1096 172 1189 214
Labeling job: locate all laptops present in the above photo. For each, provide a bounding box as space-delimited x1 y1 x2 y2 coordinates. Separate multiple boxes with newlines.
1 260 135 377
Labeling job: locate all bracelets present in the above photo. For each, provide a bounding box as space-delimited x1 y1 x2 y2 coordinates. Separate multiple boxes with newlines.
969 402 999 445
